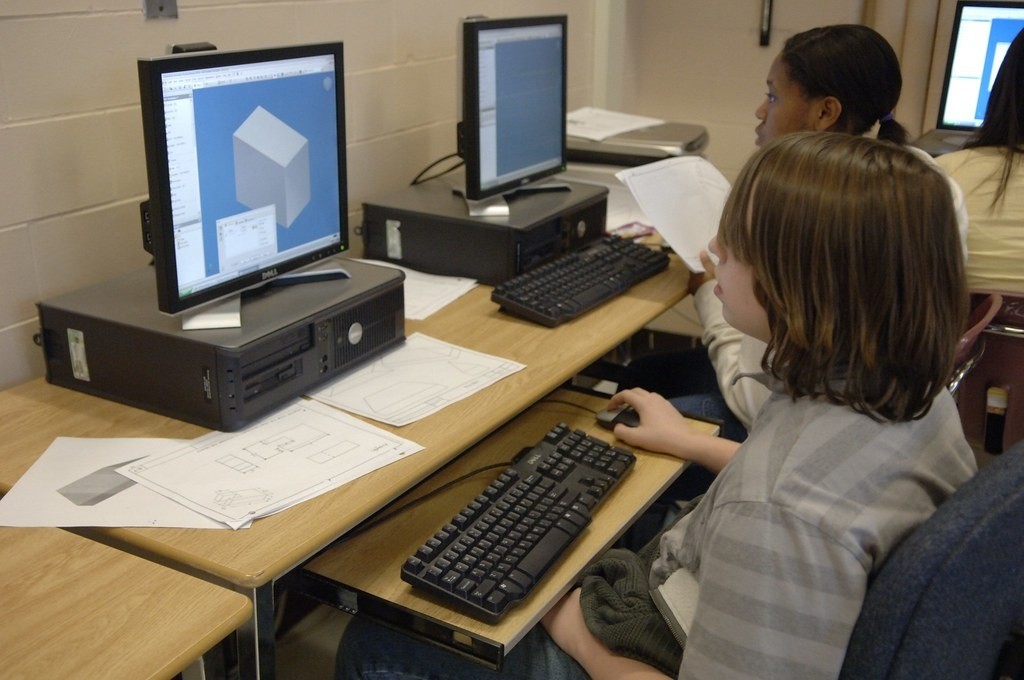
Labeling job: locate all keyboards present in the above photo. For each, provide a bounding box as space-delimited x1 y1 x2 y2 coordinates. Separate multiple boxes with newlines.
400 423 637 622
491 234 671 328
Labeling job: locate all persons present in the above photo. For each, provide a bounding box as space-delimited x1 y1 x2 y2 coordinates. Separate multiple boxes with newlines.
613 24 906 553
335 132 978 680
934 28 1024 298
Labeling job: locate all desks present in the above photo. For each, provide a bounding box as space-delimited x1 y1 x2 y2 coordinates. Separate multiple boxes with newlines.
2 526 253 679
1 237 728 672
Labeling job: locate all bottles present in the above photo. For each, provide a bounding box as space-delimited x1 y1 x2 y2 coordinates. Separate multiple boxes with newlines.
983 377 1010 455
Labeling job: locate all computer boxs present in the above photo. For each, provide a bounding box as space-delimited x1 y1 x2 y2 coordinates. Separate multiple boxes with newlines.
36 255 406 433
361 176 609 288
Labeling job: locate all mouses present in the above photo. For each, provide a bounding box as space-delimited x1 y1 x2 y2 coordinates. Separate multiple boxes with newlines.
596 406 640 431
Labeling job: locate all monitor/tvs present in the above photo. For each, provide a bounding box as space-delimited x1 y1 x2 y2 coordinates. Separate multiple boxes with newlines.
937 0 1024 131
453 14 572 216
138 41 350 330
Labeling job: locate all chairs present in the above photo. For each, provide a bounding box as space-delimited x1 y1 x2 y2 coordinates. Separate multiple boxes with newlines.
942 291 1003 394
952 289 1024 470
836 436 1024 680
